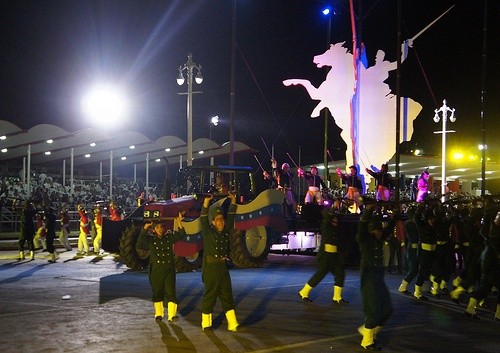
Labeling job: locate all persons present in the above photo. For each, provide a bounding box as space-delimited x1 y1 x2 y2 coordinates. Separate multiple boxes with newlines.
321 171 500 320
365 163 394 201
298 166 329 204
172 167 254 202
138 212 187 324
0 174 157 263
334 165 365 202
354 201 399 353
263 169 278 191
272 160 295 218
297 202 350 306
199 192 244 334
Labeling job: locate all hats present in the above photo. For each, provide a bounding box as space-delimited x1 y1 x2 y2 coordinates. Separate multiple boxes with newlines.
210 206 224 220
366 214 380 231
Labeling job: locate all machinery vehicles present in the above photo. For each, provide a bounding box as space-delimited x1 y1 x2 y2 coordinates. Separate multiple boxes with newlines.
101 165 286 273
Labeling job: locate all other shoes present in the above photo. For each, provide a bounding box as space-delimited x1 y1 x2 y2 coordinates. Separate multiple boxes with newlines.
439 287 500 322
360 341 386 353
156 316 163 324
229 324 248 334
297 292 313 304
428 293 443 301
168 316 179 324
203 326 214 336
401 289 411 296
413 295 429 301
332 299 349 305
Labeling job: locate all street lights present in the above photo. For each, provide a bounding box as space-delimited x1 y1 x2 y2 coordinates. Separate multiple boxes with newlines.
433 99 458 201
322 6 336 186
175 53 204 167
